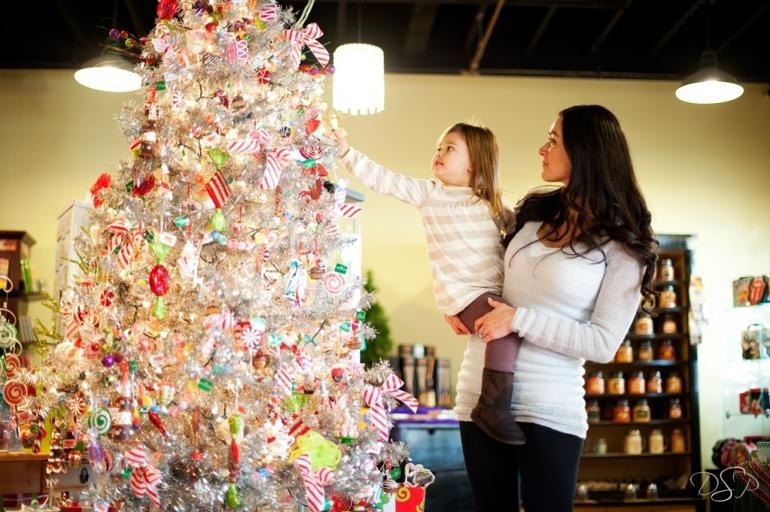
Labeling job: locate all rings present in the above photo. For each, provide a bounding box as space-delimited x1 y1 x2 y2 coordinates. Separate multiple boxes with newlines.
477 331 484 337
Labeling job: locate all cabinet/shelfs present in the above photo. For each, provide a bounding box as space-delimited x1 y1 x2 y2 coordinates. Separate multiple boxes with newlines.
572 248 703 511
1 450 48 510
393 421 477 512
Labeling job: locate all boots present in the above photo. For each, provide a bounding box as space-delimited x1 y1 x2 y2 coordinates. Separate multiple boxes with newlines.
469 365 528 447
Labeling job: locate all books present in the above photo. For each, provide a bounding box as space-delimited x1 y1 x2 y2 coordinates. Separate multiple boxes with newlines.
1 236 36 343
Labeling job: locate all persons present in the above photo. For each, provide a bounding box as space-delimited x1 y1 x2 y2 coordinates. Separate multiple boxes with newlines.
330 121 528 447
444 103 660 512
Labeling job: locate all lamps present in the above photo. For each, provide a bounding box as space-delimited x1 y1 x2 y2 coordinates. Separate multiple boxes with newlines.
71 1 141 94
673 45 744 105
332 0 386 116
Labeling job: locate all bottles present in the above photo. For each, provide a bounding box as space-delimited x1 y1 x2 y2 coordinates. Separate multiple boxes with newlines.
582 255 686 457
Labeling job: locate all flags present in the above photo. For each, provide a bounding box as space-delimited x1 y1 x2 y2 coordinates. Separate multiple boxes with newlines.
19 0 436 512
